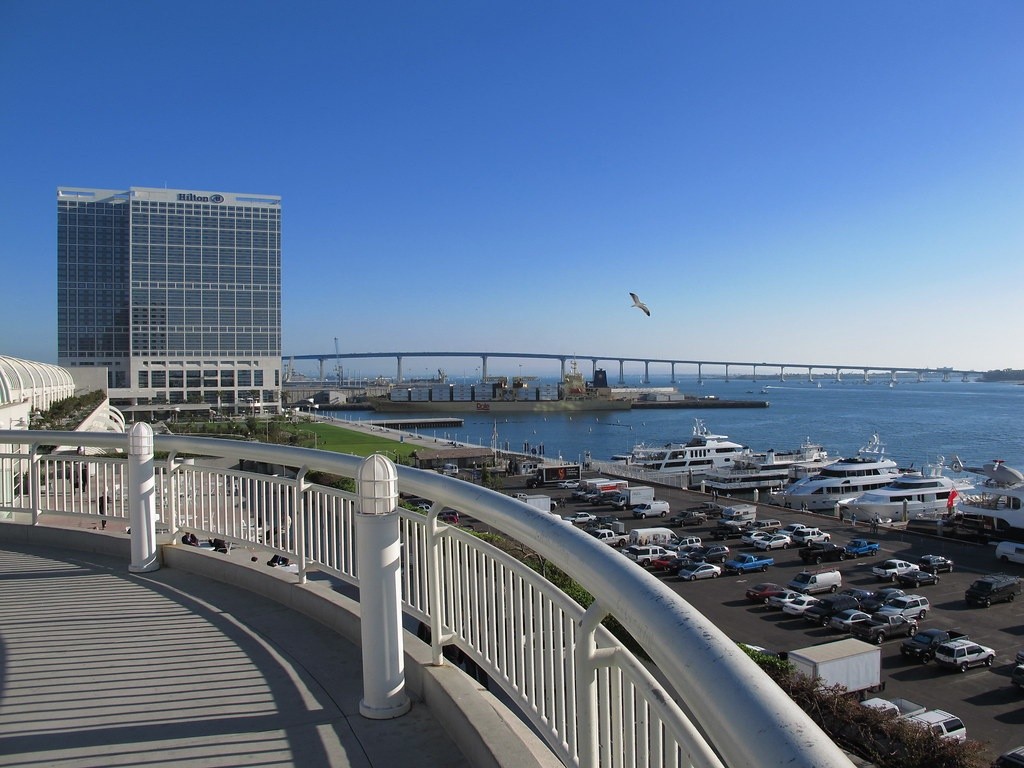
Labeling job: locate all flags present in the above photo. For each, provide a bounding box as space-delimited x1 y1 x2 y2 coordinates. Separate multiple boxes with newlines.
947 488 957 507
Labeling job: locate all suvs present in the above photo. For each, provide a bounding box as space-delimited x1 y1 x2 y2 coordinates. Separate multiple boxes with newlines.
689 545 730 563
860 589 906 614
879 594 930 619
902 709 967 749
919 555 953 575
793 528 831 544
935 640 996 673
803 597 860 626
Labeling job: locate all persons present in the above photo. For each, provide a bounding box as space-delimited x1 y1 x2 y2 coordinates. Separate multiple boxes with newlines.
840 511 844 523
82 465 88 492
870 518 878 534
182 532 196 546
712 490 718 501
851 513 856 526
99 491 109 514
271 554 289 566
208 537 227 553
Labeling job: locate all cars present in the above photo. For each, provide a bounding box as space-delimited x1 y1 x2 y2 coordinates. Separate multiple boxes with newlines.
563 511 597 524
783 596 817 616
667 558 692 574
837 588 873 602
678 562 721 581
653 554 676 569
558 480 578 489
745 583 786 603
754 535 792 551
832 609 872 632
741 530 768 545
768 589 803 610
898 570 940 588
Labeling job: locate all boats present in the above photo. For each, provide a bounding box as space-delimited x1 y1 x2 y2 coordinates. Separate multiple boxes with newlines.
767 429 921 510
702 431 846 493
611 417 755 489
941 456 1024 532
837 455 984 522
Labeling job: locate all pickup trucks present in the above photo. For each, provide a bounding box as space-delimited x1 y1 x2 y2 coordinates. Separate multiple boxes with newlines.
671 511 707 527
852 611 918 644
724 553 774 575
900 628 969 664
709 522 745 540
799 543 846 564
844 539 880 559
778 523 808 538
687 502 725 517
621 544 678 566
860 696 928 723
872 559 920 582
593 529 631 547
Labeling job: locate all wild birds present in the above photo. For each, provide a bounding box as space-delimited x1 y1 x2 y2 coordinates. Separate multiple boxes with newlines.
629 291 651 317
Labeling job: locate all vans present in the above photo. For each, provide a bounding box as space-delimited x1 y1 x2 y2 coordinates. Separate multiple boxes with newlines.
632 501 670 519
788 568 842 595
748 519 782 534
630 528 677 546
996 541 1024 565
669 535 702 551
592 491 618 505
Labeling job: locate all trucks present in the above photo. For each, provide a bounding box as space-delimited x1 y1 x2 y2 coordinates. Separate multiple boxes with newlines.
966 575 1021 608
612 486 655 511
583 480 628 503
571 477 609 498
